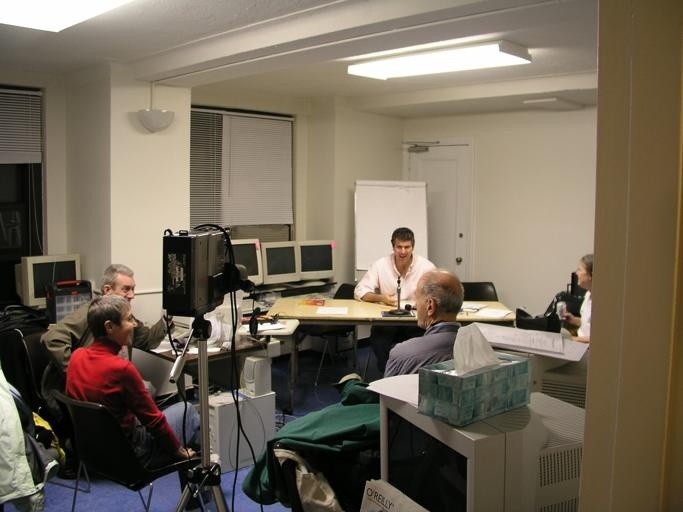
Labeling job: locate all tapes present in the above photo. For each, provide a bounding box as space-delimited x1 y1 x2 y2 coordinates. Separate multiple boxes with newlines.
535 315 545 318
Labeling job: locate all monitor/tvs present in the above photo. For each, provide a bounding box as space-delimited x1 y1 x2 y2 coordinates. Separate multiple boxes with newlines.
230 239 263 295
380 390 586 512
297 239 335 286
261 241 297 291
15 253 81 310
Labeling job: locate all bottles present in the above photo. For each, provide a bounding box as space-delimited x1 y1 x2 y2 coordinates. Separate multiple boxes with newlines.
556 301 567 321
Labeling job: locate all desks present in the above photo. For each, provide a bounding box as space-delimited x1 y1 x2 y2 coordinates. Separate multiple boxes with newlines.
486 334 586 410
124 314 301 453
266 294 519 387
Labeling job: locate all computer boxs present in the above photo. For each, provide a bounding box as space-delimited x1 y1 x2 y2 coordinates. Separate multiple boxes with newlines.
208 389 276 473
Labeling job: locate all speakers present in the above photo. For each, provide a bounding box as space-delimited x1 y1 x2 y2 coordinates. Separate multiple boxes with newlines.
244 356 272 397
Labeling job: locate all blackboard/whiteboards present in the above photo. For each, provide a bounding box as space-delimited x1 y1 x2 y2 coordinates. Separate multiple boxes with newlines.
353 180 428 284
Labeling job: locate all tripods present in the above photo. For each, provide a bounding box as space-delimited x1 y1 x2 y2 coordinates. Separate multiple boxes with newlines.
169 317 229 511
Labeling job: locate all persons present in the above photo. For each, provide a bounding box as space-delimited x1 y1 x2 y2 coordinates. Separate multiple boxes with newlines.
382 269 465 379
352 228 438 374
64 293 213 510
555 254 594 343
38 263 174 404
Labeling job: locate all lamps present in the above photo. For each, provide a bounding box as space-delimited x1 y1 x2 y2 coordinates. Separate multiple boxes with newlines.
138 105 176 135
341 36 535 83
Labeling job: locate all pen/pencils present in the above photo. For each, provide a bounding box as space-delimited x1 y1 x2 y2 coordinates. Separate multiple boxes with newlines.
397 274 401 310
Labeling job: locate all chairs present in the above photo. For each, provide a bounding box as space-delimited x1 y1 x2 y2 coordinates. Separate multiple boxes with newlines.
361 333 378 373
45 387 205 512
11 324 100 496
297 280 364 385
457 280 496 301
